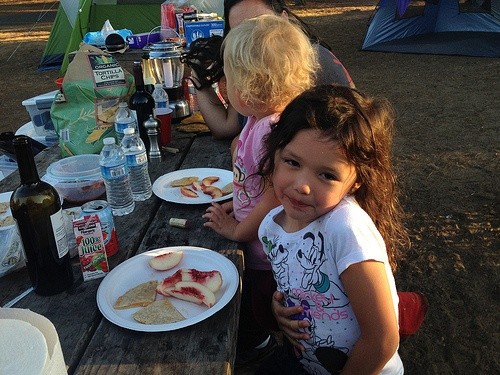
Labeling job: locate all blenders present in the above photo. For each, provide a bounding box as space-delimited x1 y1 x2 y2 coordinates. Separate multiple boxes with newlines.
141 24 191 122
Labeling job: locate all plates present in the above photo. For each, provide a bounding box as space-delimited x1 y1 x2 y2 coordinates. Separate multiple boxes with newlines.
152 168 235 204
96 245 239 332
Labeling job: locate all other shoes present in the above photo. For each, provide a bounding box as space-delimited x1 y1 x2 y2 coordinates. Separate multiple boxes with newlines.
235 329 278 367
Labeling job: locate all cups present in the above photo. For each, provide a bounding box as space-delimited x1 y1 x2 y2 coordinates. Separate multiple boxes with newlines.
156 108 173 146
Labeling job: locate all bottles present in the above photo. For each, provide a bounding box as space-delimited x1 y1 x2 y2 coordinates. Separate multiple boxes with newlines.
98 63 169 216
10 136 75 294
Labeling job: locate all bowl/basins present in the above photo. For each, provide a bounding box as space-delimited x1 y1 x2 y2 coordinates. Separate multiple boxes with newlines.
47 154 107 202
0 191 26 269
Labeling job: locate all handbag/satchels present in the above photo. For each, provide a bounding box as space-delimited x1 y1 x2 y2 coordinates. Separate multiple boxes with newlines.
50 44 137 157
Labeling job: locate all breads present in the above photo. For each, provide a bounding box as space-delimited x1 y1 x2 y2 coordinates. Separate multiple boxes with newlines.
0 202 14 227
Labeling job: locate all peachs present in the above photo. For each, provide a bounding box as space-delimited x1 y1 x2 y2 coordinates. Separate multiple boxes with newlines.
148 251 222 307
181 176 234 199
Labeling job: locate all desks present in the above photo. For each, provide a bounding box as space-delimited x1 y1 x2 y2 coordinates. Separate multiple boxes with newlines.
68 48 188 79
0 131 250 375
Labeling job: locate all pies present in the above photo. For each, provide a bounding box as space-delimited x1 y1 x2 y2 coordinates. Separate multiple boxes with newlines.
114 280 158 309
170 176 199 187
133 299 185 325
178 111 210 134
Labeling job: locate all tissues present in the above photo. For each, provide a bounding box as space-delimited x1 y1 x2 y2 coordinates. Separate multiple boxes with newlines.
82 18 133 47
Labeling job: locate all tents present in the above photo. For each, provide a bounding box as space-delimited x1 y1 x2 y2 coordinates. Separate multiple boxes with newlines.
357 0 500 57
38 0 169 77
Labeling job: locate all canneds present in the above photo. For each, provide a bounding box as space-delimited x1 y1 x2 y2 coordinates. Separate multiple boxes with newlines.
160 3 178 39
80 200 118 258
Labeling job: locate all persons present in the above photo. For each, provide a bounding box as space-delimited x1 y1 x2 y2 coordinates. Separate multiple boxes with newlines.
202 12 321 361
191 0 358 140
236 83 412 375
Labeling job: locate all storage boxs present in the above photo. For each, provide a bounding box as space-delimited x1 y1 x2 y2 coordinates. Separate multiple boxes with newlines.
126 31 161 49
185 19 224 49
21 90 63 136
